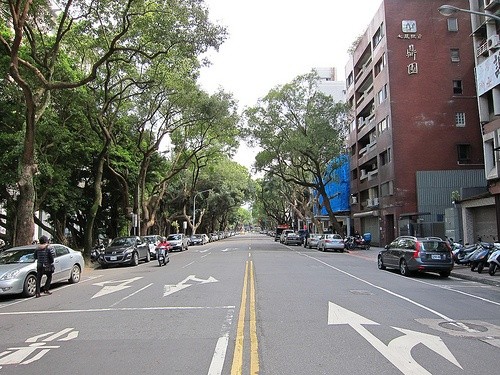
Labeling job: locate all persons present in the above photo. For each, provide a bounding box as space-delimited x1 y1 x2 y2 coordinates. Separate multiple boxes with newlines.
155 237 170 257
353 230 360 240
33 235 54 298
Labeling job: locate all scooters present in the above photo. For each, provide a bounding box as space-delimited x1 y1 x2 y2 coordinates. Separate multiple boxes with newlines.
344 233 370 250
486 243 500 276
474 237 500 274
445 236 476 271
81 242 105 264
154 243 170 267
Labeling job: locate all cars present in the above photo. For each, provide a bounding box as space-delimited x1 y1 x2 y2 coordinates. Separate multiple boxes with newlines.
285 233 301 246
0 243 85 297
259 230 276 237
208 230 240 242
317 234 344 253
189 234 211 246
303 234 322 249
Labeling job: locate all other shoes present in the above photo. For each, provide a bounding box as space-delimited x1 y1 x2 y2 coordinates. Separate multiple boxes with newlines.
44 290 52 294
36 293 40 297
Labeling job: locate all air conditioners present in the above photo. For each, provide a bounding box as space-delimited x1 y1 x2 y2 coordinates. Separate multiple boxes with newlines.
486 35 500 49
360 170 366 177
366 199 372 208
484 0 499 10
372 198 379 206
351 196 357 205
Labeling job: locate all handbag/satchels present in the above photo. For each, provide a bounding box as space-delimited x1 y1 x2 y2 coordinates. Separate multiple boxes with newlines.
45 264 55 273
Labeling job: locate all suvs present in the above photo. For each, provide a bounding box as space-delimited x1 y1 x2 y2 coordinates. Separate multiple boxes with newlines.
97 236 151 268
166 233 189 253
275 227 289 242
378 236 454 277
279 230 294 244
141 235 170 260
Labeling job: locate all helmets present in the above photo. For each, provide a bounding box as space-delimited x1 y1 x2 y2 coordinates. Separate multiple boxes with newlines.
161 236 167 243
